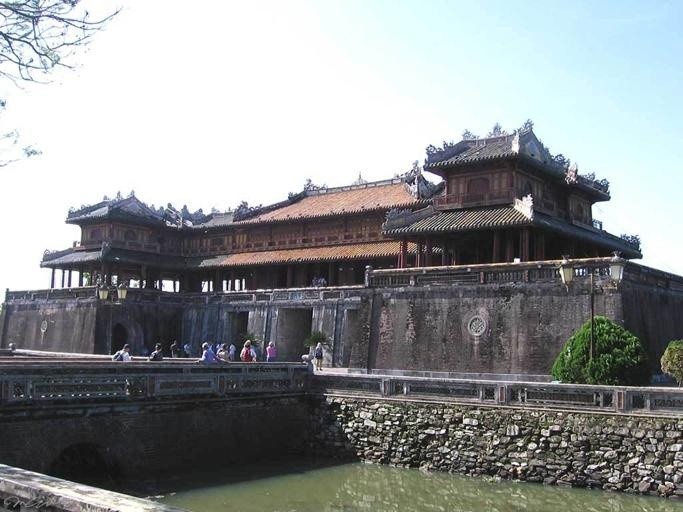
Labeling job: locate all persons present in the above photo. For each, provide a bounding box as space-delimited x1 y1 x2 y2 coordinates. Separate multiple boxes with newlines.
265 341 278 362
148 343 163 361
314 342 325 371
169 339 258 362
111 344 133 362
309 273 328 287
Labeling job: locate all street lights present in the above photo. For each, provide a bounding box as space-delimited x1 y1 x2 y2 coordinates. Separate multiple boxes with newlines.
98 282 128 355
556 250 626 359
